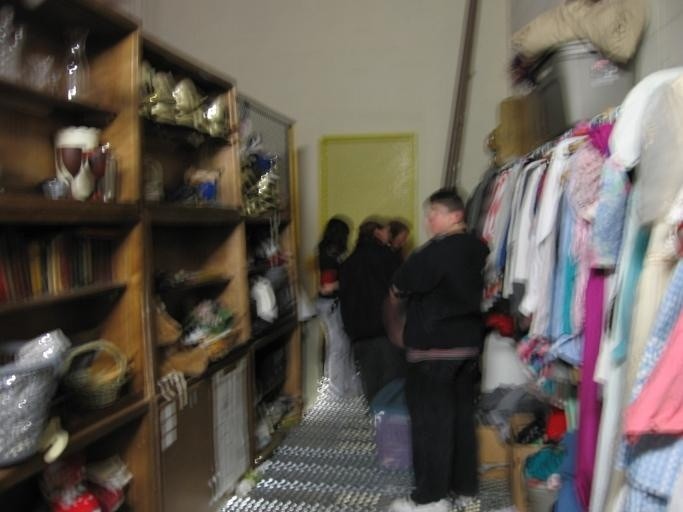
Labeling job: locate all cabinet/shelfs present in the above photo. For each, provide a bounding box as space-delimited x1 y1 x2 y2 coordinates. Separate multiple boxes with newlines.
0 0 304 512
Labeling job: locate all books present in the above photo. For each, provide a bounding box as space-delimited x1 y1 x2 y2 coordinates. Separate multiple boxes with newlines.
1 232 113 304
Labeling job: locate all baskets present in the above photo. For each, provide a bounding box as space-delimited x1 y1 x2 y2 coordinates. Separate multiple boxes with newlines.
58 339 131 408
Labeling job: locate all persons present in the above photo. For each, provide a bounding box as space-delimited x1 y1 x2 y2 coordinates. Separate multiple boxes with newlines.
386 185 493 512
298 212 362 400
337 214 403 415
383 215 415 271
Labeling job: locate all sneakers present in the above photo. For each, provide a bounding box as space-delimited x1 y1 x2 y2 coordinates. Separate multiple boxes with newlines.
386 492 454 512
447 493 482 512
